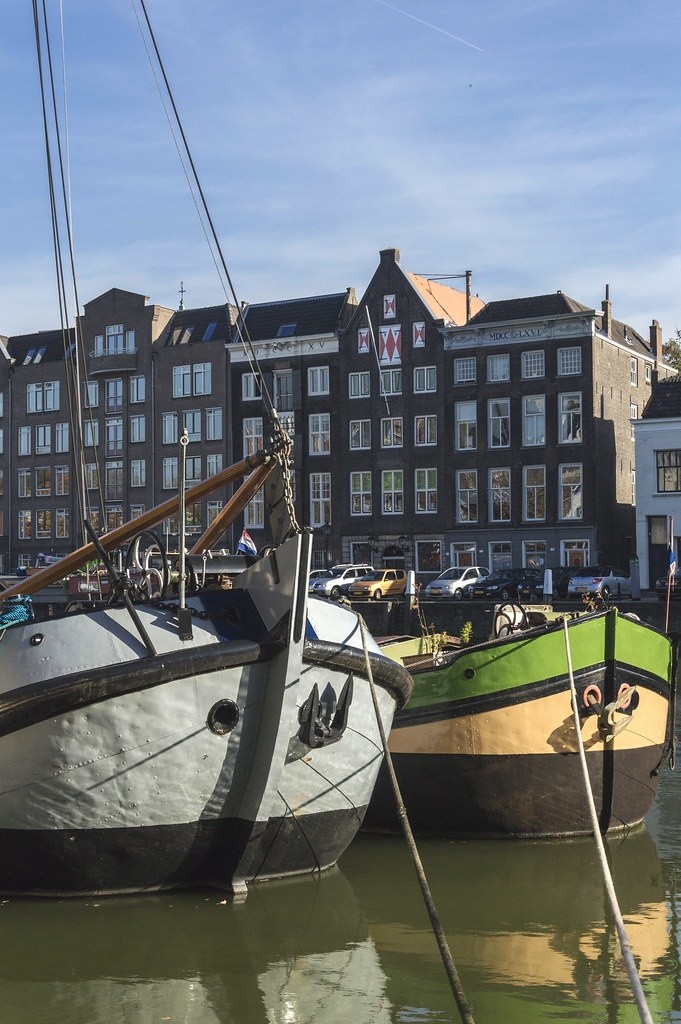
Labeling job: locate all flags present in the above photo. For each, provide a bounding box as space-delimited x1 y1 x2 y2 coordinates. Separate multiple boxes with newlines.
238 530 258 556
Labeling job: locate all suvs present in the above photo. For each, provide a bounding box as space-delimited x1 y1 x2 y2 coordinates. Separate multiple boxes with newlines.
304 561 634 605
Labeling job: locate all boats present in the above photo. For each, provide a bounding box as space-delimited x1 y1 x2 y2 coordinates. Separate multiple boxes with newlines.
345 596 677 843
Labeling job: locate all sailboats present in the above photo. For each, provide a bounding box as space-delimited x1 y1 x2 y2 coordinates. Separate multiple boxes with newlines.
0 0 407 904
